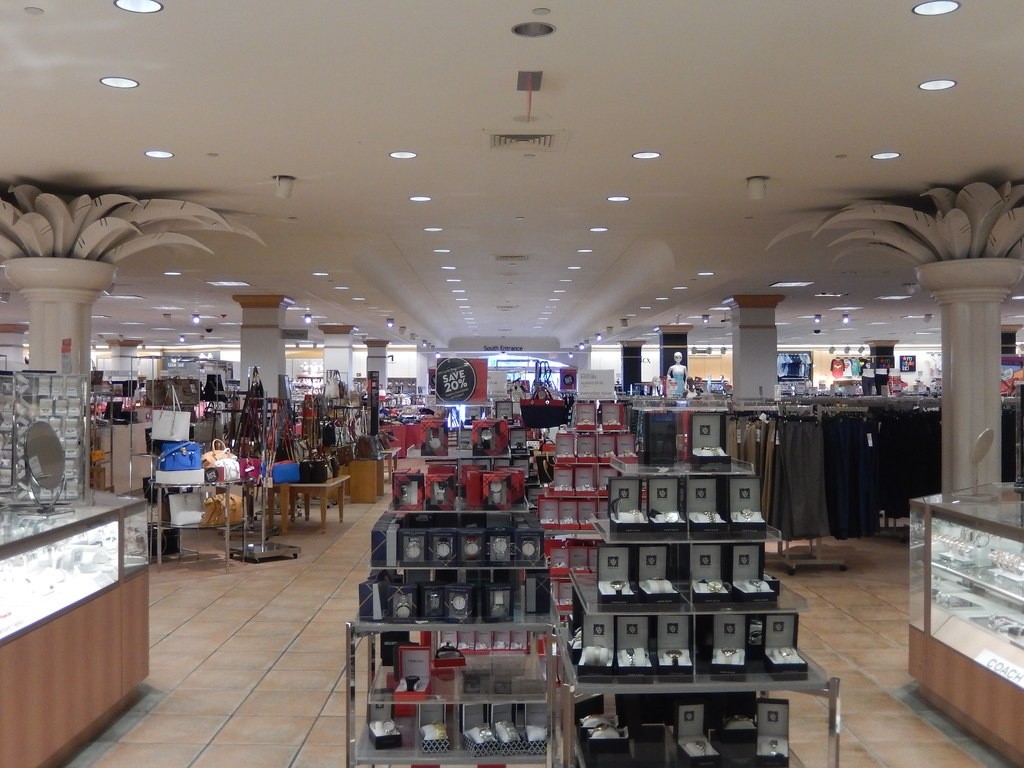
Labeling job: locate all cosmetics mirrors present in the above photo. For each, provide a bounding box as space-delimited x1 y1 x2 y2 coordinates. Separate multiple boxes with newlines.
957 428 999 502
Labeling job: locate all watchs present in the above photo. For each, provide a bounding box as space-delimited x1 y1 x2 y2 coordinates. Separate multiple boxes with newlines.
381 719 519 741
706 578 763 593
694 739 706 756
702 445 721 456
610 575 668 594
399 481 504 505
404 675 421 691
590 723 618 736
407 535 539 561
592 644 682 666
704 509 755 522
721 646 794 664
396 592 469 617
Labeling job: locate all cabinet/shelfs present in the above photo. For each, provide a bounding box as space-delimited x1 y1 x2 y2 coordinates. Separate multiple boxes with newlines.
347 398 559 768
542 395 639 686
505 428 540 514
555 400 840 767
0 370 114 507
908 481 1024 768
293 374 323 389
129 354 253 573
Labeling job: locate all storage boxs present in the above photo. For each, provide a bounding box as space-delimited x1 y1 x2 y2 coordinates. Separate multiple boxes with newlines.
156 469 204 484
369 401 808 767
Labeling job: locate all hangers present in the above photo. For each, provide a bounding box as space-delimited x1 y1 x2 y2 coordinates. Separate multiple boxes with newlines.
745 400 1016 425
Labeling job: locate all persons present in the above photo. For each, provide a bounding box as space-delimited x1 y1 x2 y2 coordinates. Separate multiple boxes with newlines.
684 376 703 399
647 376 662 397
874 362 890 396
859 358 875 396
667 351 688 398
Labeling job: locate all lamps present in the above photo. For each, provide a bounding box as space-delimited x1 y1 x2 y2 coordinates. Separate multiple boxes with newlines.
164 175 932 359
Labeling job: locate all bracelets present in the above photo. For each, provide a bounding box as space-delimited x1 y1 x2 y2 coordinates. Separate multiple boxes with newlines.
770 739 777 755
937 534 1021 574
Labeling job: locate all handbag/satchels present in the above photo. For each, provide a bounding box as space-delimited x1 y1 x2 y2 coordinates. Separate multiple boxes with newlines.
216 460 240 480
145 379 200 405
202 439 238 467
336 436 381 466
151 385 191 441
160 441 201 471
239 458 260 478
520 385 567 428
300 452 339 484
169 492 213 525
203 493 243 524
194 413 223 442
204 374 227 402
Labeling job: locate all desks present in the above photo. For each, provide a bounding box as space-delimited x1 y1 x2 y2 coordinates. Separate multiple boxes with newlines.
266 424 425 534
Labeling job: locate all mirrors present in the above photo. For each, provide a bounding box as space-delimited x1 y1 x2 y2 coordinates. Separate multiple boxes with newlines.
23 422 66 513
958 428 998 503
